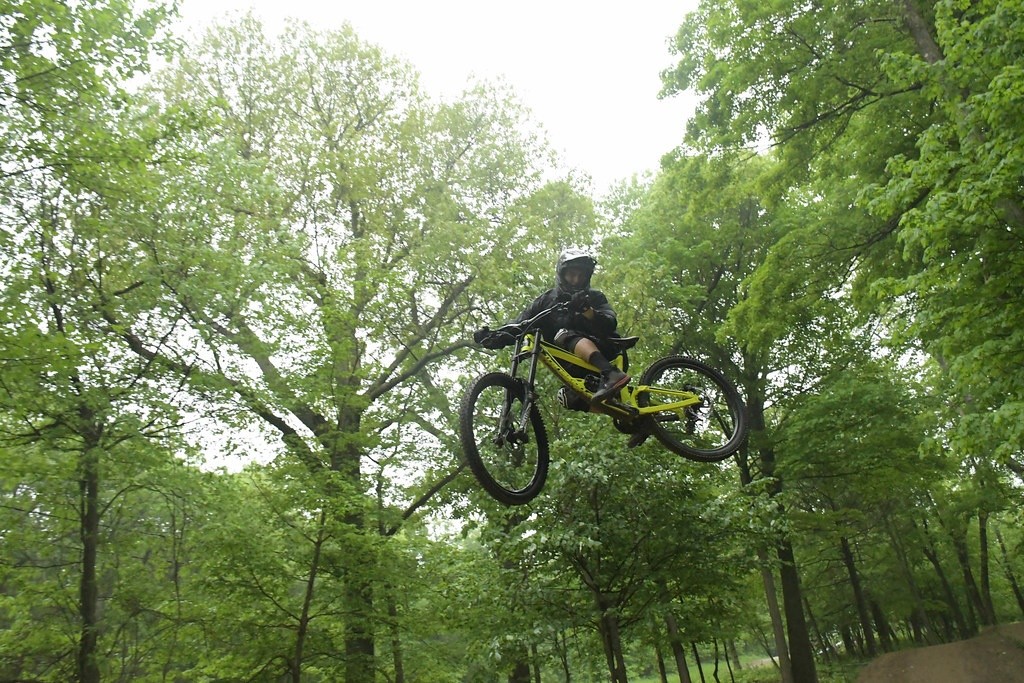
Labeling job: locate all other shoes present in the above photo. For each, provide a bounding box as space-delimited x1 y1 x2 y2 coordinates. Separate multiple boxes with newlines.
627 431 647 447
591 365 631 404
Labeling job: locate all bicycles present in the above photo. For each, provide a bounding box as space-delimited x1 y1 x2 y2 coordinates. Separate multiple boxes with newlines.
459 291 751 506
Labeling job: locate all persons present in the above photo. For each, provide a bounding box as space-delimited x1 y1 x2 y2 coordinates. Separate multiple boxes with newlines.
474 249 632 413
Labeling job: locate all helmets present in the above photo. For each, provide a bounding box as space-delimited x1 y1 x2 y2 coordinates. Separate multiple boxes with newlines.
557 248 598 298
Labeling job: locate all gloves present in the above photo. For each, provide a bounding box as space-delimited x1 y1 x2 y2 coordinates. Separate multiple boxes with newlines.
570 291 590 314
473 325 492 344
